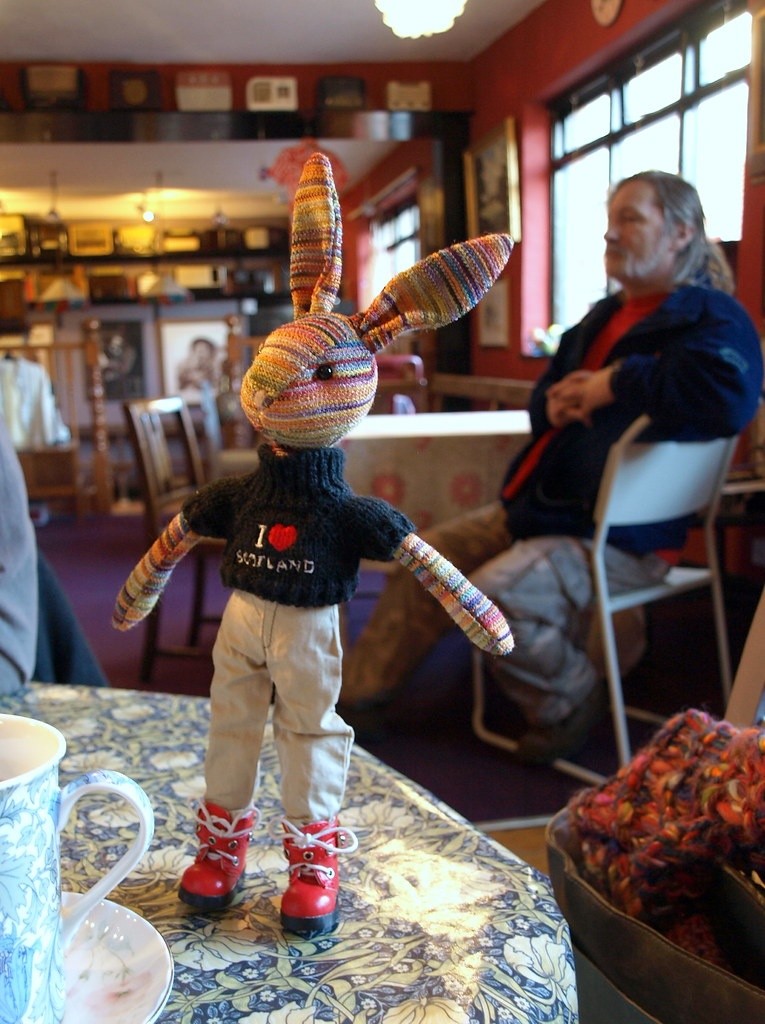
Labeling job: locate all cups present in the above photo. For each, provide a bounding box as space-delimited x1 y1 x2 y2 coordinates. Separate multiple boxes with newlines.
0 714 155 1024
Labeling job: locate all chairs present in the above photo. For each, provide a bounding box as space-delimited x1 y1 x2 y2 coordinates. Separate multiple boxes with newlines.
122 394 232 685
468 413 742 794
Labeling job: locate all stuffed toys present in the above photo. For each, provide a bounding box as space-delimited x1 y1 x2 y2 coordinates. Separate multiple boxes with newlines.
111 154 519 933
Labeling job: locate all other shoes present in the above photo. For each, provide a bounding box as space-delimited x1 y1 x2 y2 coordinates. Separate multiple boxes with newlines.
515 682 606 771
334 699 385 743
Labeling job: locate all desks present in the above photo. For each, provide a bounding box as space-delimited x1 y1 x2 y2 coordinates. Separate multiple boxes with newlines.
0 677 584 1024
316 405 544 703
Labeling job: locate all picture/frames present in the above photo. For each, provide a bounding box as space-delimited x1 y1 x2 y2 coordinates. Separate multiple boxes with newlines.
155 314 238 405
63 303 156 423
461 115 522 246
475 275 514 349
67 219 114 257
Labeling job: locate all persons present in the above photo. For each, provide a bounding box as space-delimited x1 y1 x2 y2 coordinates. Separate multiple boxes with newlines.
334 170 762 774
0 435 109 696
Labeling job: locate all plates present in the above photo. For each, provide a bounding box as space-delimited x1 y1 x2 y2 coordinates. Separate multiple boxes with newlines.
60 890 175 1024
